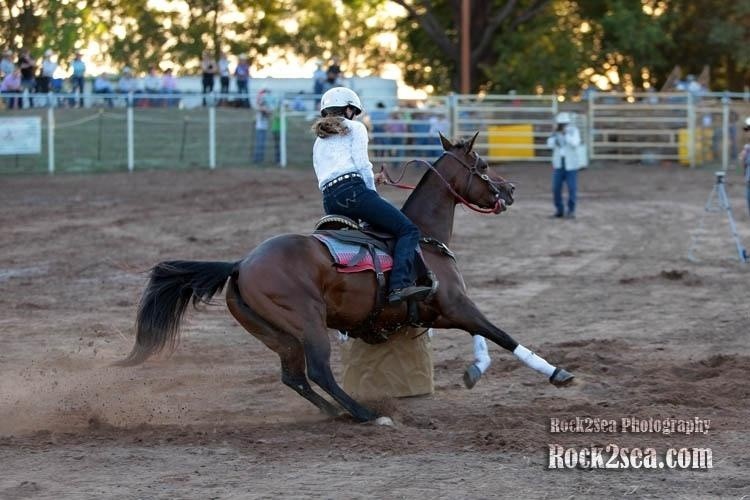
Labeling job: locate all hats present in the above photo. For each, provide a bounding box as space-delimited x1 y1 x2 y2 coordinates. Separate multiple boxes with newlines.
557 112 570 123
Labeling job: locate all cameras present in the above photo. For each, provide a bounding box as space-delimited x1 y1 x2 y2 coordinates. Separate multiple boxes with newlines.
556 123 566 131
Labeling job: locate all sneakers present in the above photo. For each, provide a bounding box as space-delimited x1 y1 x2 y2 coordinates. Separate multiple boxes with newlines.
548 211 575 220
389 286 432 304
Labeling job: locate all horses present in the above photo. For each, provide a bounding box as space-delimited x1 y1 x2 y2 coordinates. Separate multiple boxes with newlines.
100 131 575 426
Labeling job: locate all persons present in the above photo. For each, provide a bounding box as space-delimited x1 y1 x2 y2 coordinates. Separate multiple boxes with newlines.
738 117 750 215
312 87 433 306
0 53 446 168
546 112 581 219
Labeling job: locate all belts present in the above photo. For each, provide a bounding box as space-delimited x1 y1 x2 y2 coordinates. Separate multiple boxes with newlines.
321 173 363 193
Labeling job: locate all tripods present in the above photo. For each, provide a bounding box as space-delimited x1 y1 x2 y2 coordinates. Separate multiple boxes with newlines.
687 172 749 263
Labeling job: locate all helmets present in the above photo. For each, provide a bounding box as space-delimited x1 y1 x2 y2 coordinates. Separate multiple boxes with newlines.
320 87 361 117
